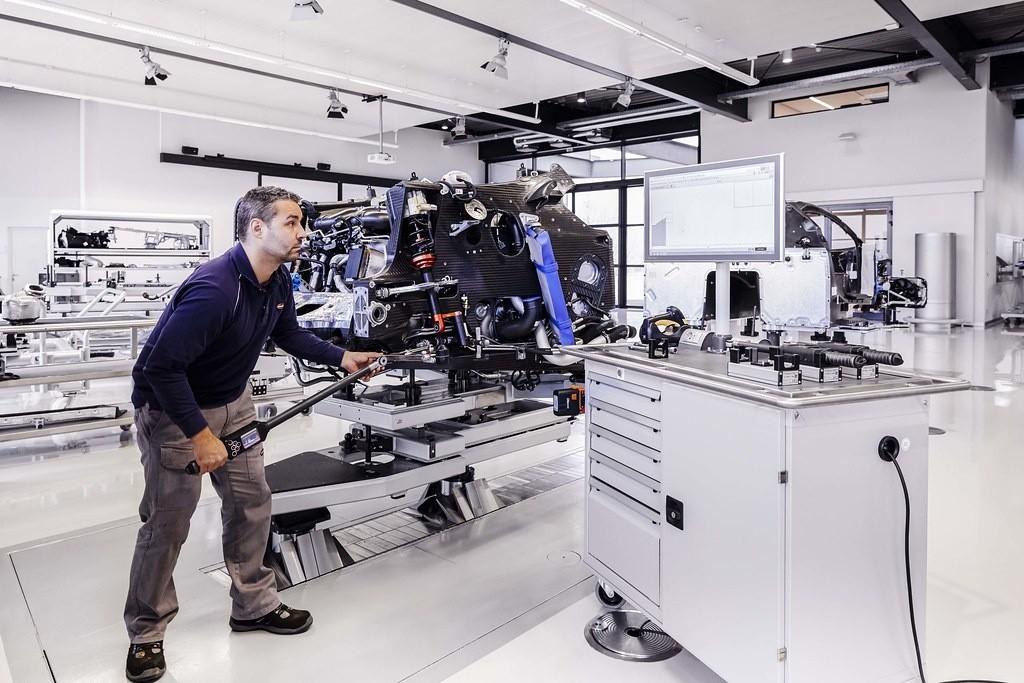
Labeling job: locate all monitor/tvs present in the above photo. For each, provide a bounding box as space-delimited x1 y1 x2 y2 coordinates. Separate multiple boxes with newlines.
644 152 786 262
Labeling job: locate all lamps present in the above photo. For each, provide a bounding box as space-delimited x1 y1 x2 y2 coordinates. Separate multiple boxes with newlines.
480 38 511 79
611 84 636 112
291 0 324 20
326 90 348 119
577 92 587 103
140 46 171 86
451 116 468 141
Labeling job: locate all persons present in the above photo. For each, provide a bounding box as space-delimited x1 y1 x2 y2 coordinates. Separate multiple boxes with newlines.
124 184 388 683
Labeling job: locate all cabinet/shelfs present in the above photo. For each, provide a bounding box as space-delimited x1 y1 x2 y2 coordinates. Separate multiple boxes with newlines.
559 343 972 683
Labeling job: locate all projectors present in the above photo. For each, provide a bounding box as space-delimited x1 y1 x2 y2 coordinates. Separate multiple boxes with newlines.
368 153 396 165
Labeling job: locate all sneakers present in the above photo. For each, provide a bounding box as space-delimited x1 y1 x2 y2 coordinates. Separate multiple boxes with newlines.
126 640 166 683
229 603 313 633
